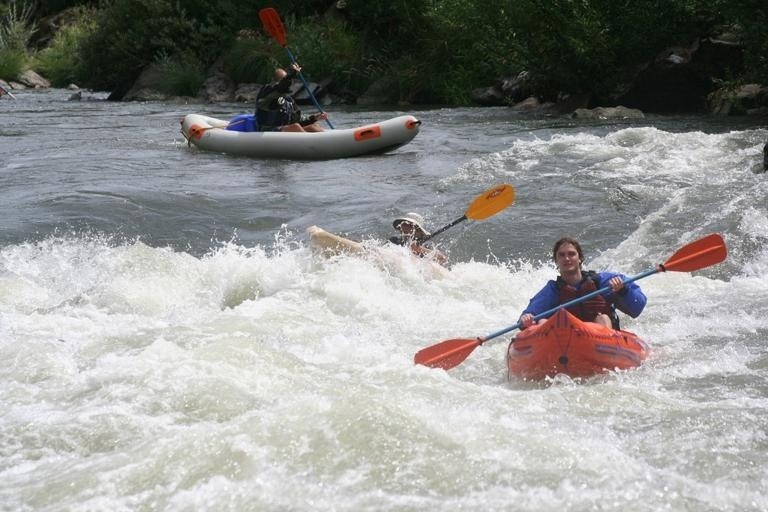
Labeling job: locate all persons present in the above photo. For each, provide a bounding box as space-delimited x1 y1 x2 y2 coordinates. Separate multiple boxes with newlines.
516 235 648 333
252 61 331 133
386 211 450 264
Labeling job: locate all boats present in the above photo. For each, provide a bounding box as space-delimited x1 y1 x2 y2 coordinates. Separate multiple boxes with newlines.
505 305 651 379
179 114 422 160
307 227 450 282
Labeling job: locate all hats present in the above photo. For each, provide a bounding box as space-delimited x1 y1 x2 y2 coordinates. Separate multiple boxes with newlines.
392 213 432 236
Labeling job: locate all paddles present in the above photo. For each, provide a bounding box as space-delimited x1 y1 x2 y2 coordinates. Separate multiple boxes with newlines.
415 234 727 371
419 183 515 245
259 8 333 129
